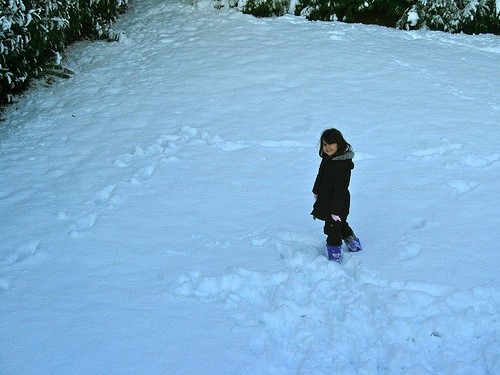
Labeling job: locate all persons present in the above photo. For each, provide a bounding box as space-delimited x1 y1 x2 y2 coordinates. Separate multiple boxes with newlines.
309 128 363 264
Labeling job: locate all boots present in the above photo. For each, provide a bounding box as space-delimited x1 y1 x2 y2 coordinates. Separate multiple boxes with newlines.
327 246 341 262
346 236 361 252
